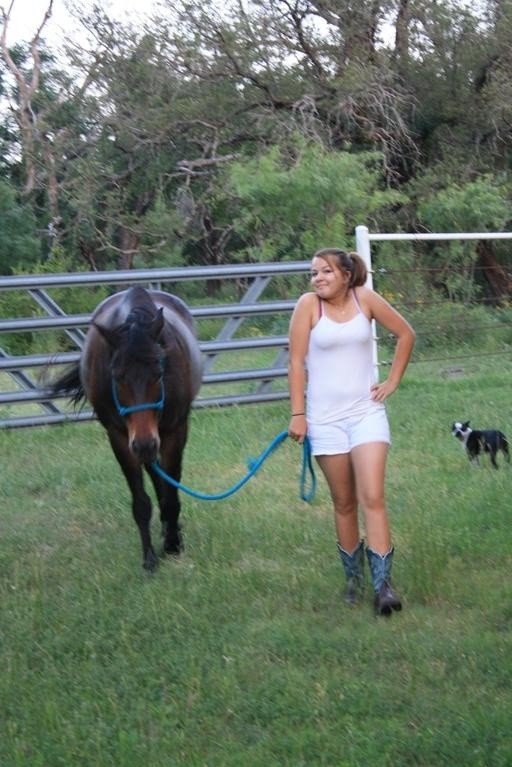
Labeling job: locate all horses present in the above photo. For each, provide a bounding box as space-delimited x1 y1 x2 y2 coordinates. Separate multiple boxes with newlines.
35 284 204 573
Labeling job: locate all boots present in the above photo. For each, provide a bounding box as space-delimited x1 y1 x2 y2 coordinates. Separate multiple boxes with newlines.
365 545 402 616
337 539 366 608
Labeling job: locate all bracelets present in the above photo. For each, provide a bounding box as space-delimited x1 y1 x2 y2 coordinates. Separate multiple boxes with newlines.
291 412 305 417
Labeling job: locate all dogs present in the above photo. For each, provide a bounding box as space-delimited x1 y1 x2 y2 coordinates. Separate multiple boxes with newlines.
451 420 511 470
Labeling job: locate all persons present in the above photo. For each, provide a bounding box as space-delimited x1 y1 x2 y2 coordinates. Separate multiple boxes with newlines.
287 247 416 615
